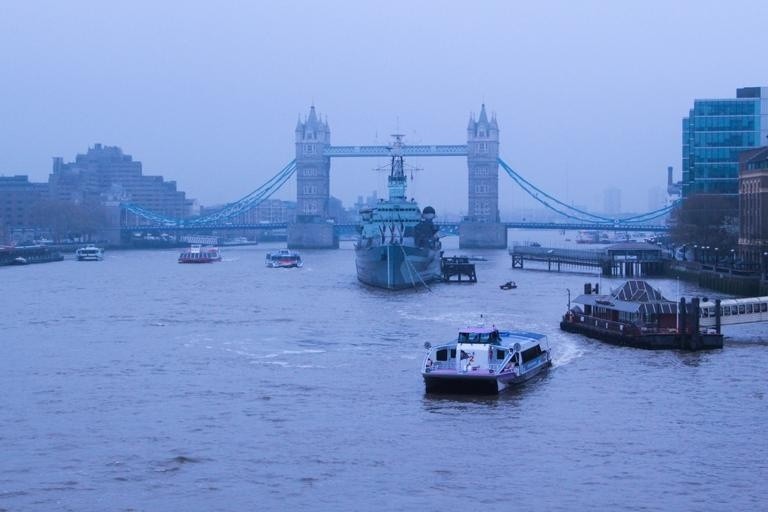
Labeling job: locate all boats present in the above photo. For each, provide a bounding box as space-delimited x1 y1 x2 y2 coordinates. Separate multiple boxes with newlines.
75 244 104 261
561 280 723 352
223 237 257 246
421 325 551 395
177 244 221 265
575 229 637 243
0 245 63 266
266 250 303 268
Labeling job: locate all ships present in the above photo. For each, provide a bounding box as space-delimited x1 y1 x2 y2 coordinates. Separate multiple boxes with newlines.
354 134 442 291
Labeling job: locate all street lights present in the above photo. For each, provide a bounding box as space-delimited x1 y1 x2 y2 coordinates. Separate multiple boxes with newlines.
657 241 735 265
